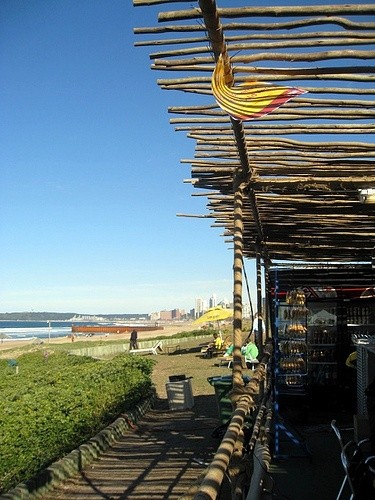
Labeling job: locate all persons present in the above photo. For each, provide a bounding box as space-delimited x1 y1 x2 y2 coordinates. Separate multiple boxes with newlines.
241 339 259 361
130 328 137 350
205 332 222 359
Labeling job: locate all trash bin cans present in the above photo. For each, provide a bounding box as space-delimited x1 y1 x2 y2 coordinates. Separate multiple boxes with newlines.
207 376 255 445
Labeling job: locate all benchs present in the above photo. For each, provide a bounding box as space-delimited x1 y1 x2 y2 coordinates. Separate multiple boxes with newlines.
251 360 259 370
218 357 233 368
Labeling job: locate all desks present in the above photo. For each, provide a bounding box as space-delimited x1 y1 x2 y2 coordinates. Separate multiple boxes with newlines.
166 345 180 354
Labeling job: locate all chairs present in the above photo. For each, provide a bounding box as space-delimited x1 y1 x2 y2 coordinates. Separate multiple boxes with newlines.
130 341 161 355
331 419 375 500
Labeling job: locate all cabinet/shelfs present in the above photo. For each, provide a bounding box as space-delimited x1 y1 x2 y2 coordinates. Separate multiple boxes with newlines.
347 323 375 346
278 301 337 396
166 379 194 410
215 382 235 418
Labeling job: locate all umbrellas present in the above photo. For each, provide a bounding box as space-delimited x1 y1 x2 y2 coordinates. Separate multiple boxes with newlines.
192 307 234 340
